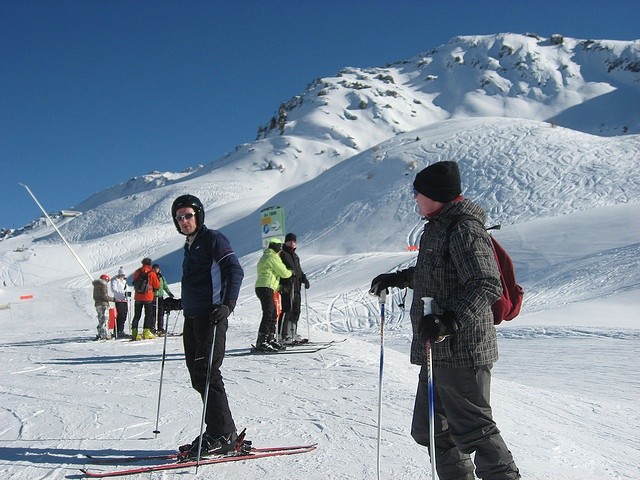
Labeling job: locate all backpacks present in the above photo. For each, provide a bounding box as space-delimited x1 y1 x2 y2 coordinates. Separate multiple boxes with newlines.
445 214 524 324
135 269 152 294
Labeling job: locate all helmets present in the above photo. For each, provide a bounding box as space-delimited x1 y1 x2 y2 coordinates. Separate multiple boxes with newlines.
172 194 204 231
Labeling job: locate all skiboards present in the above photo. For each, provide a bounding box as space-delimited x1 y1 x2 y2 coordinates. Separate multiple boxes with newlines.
248 343 332 354
79 443 318 478
160 331 183 337
279 338 347 346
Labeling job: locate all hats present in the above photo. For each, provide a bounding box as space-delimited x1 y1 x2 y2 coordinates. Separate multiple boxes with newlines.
100 275 110 281
118 266 125 276
413 161 462 203
285 233 297 242
269 239 281 244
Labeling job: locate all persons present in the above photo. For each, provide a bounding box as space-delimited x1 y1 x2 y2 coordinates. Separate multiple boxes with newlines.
172 194 244 461
152 264 174 334
91 275 116 339
111 265 131 339
254 238 295 355
370 161 522 476
277 233 310 345
132 257 159 340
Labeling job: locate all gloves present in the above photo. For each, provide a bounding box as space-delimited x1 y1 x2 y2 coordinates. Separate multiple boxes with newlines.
418 311 454 344
161 298 181 314
208 304 230 327
302 277 309 288
370 267 415 297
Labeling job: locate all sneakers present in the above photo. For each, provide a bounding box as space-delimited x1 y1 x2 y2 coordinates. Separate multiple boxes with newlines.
179 432 206 452
143 328 156 339
131 327 143 340
179 432 241 460
278 336 299 347
256 333 278 351
267 333 286 350
290 334 308 344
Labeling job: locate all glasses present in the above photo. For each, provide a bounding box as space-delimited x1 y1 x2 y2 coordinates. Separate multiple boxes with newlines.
414 188 419 195
175 211 195 221
